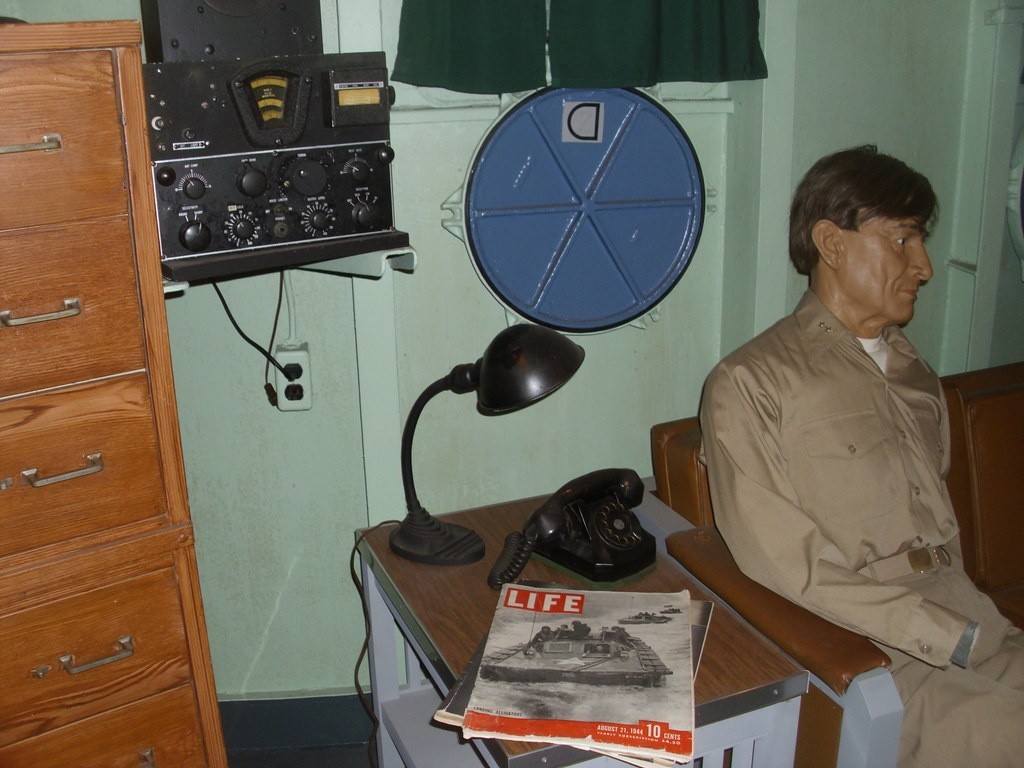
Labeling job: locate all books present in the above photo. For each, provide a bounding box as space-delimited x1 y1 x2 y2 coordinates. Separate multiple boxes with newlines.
433 600 715 768
464 583 696 762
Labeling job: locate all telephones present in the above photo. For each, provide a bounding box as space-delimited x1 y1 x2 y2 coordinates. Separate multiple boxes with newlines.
522 466 660 585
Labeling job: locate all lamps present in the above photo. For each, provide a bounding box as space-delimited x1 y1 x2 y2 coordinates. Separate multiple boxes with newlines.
391 322 586 565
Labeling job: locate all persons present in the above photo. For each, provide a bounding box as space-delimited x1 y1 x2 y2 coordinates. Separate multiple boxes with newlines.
700 146 1024 767
531 620 591 642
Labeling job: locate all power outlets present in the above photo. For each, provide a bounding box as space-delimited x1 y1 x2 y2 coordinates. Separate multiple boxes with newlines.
275 342 313 411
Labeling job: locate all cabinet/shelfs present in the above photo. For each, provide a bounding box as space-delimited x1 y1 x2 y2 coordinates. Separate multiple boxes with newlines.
355 483 814 768
1 19 230 767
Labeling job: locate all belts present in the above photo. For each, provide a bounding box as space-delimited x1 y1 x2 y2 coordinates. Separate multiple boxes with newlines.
858 545 949 584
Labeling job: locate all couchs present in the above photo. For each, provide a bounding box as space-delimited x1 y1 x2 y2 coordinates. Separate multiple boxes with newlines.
637 361 1024 767
